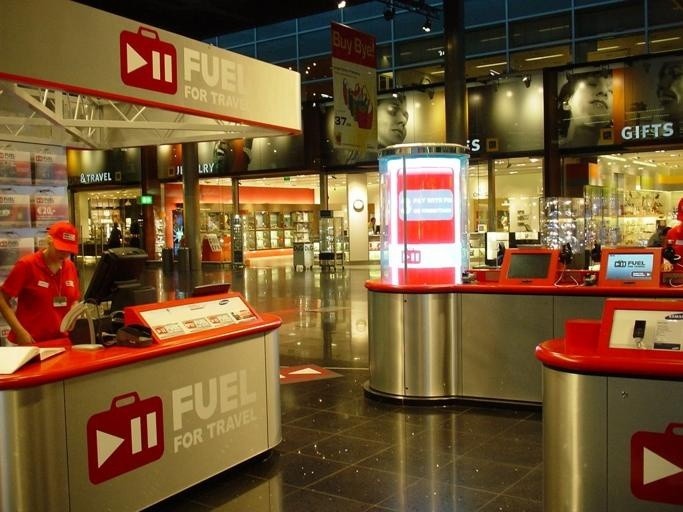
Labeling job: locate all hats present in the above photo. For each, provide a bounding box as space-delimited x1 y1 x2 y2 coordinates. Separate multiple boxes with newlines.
677 198 683 221
48 221 80 255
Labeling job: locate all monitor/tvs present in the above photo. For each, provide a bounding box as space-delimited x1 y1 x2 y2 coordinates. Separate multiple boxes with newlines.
82 247 156 318
598 248 662 288
499 248 559 286
597 298 683 364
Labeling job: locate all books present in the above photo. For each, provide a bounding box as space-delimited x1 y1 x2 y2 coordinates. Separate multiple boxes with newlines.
0 346 65 375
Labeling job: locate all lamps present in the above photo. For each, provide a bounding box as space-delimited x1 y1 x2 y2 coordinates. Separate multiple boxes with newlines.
383 5 432 33
522 74 532 88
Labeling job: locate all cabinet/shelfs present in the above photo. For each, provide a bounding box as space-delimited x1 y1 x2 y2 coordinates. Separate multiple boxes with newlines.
583 184 683 247
173 205 314 259
318 210 346 273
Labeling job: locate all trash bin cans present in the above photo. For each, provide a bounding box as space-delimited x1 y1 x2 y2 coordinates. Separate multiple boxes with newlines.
292 242 312 272
179 247 189 264
162 248 172 272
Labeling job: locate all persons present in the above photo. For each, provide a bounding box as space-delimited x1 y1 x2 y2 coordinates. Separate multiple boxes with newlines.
108 222 122 248
557 69 614 148
0 221 81 346
359 98 408 162
664 198 683 274
656 60 683 140
368 218 376 232
